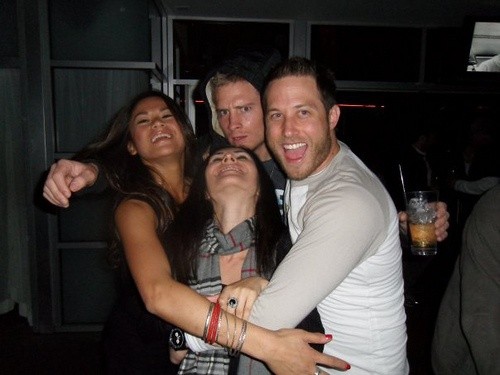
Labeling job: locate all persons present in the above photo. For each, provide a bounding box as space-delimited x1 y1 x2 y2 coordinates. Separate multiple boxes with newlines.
167 145 326 375
167 56 412 375
429 182 500 375
370 115 500 239
44 54 451 246
89 88 352 374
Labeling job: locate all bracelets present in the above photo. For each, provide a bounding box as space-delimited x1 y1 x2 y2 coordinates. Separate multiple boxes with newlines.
201 300 249 359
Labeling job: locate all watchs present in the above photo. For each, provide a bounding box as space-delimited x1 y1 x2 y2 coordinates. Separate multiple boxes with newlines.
168 327 192 352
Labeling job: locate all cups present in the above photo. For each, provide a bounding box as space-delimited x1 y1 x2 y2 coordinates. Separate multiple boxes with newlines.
403 190 439 257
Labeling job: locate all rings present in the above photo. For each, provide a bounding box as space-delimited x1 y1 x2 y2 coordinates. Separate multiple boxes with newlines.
228 297 239 309
316 368 321 375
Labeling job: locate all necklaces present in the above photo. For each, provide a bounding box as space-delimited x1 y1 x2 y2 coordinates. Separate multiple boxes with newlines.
269 164 278 177
307 168 327 176
